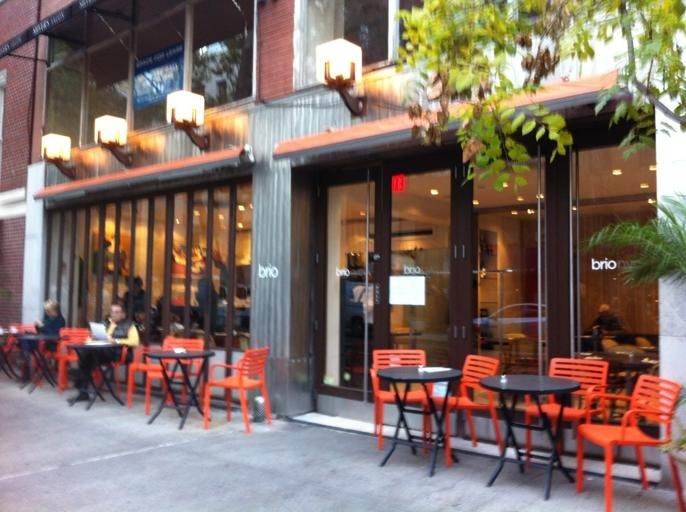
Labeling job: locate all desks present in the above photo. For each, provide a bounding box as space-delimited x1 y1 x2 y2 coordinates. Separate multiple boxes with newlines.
377 366 459 476
479 374 579 500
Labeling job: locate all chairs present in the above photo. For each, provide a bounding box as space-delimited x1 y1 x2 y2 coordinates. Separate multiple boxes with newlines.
576 375 685 511
525 358 608 493
423 355 505 466
370 349 434 454
3 320 272 433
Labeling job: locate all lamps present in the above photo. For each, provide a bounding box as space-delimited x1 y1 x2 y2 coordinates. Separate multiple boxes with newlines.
39 132 76 181
165 88 210 151
314 37 368 117
93 114 135 168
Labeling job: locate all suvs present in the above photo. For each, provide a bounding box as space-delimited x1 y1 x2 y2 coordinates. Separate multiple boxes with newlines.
343 279 373 337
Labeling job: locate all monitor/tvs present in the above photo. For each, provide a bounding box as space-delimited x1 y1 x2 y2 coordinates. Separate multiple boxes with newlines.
344 277 375 308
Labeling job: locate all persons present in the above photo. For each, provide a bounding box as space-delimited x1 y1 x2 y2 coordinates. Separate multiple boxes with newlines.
593 305 628 354
23 275 251 402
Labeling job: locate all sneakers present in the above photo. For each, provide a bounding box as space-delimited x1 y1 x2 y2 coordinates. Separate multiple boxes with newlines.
68 392 90 403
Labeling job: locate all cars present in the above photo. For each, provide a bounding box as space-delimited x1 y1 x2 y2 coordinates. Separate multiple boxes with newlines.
474 301 547 344
